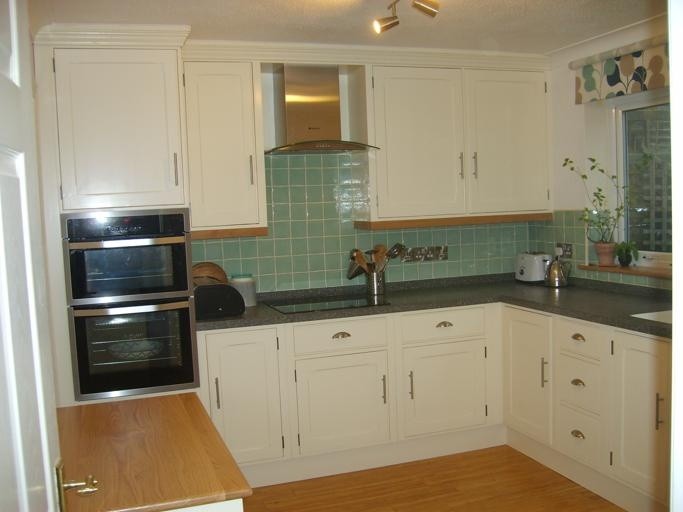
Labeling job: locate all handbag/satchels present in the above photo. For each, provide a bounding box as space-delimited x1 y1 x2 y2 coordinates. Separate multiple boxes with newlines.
194 284 245 319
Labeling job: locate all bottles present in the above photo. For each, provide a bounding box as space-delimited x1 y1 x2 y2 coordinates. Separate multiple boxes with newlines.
229 273 259 307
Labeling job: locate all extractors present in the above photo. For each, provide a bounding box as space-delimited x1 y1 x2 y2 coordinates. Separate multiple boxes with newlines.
263 64 382 159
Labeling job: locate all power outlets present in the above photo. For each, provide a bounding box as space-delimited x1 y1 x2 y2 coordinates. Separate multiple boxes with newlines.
557 243 573 258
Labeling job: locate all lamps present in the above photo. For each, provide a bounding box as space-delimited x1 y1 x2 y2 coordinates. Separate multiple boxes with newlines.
373 0 440 34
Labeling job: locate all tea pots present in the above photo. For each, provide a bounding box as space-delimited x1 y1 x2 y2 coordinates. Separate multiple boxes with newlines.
544 255 573 289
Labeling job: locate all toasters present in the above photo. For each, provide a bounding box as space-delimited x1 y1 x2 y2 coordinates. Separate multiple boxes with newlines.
512 250 553 286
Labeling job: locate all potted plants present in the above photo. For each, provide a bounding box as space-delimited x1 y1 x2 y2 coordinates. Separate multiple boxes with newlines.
562 152 659 267
613 241 640 267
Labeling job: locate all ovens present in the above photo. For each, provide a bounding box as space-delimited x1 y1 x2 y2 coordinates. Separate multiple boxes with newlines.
58 207 198 402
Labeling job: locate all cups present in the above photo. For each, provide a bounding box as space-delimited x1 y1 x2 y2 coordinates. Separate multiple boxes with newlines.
366 271 387 296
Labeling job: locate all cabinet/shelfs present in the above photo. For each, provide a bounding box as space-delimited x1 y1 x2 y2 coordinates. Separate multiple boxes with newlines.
181 43 268 238
33 23 189 214
57 393 254 512
502 303 552 474
196 327 289 488
284 301 502 485
349 49 553 230
610 327 672 512
552 315 610 501
